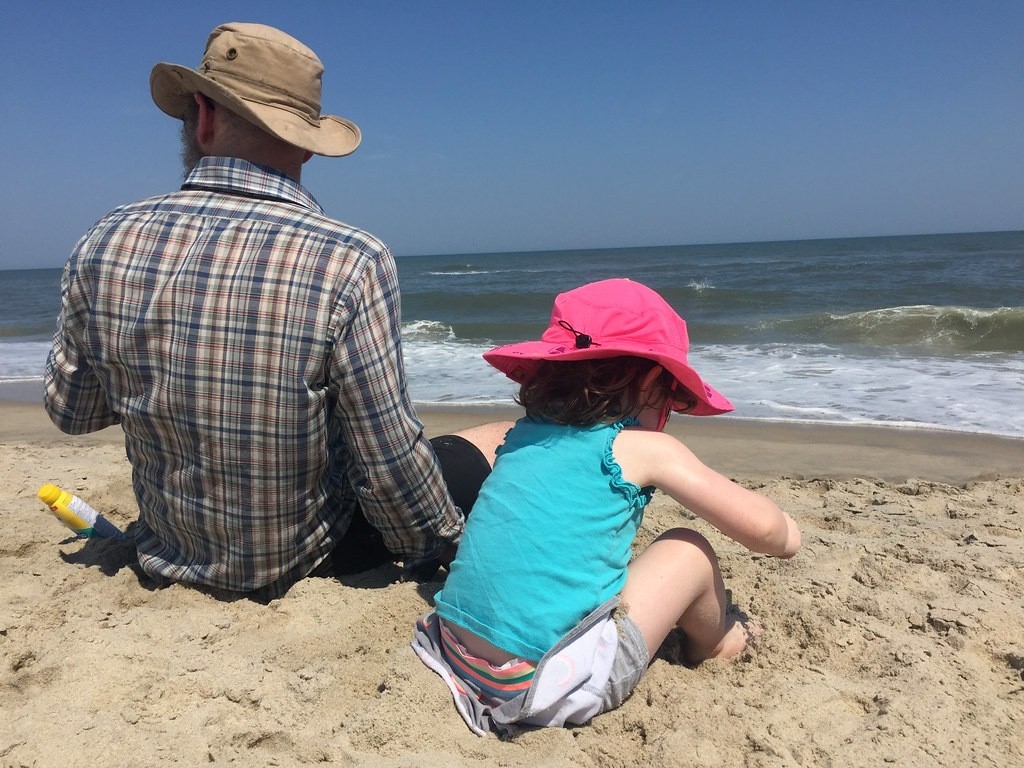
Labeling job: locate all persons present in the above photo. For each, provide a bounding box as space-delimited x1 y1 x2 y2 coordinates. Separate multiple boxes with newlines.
40 19 517 605
433 274 803 726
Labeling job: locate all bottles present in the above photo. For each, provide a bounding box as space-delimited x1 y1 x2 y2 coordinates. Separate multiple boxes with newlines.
37 484 123 539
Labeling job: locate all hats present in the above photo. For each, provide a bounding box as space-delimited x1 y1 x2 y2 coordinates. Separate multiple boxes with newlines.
150 21 361 157
482 277 735 416
